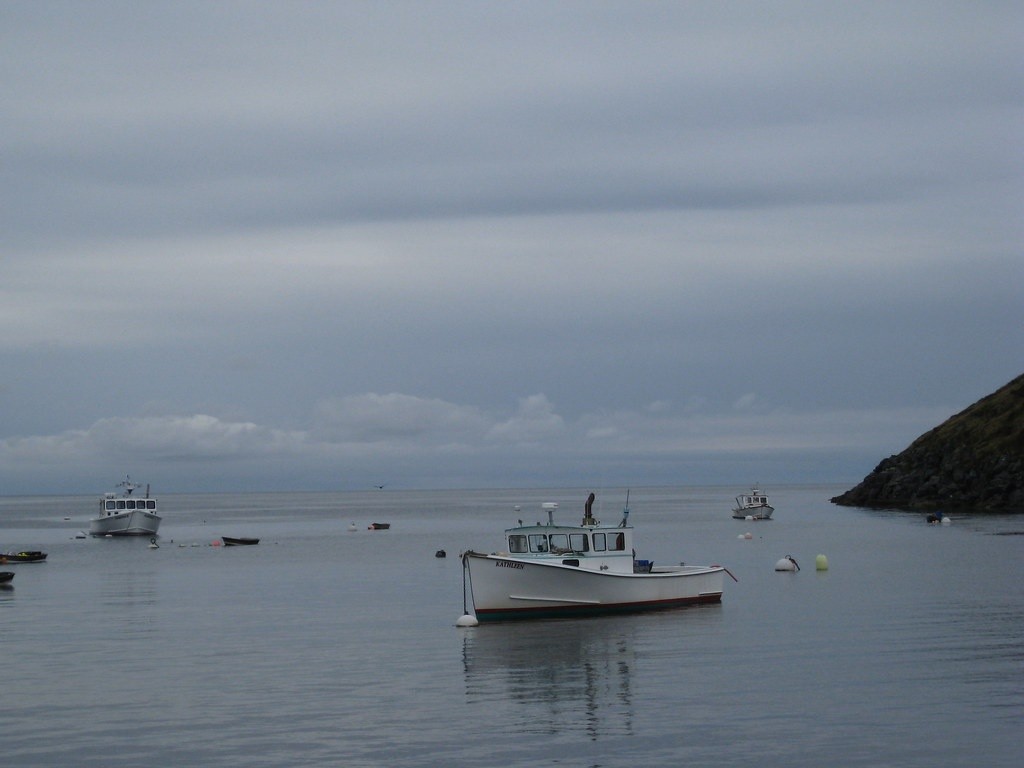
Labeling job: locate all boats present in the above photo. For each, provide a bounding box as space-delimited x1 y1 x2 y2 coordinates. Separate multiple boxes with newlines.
0 550 50 563
731 485 776 520
458 489 739 626
221 535 260 547
0 571 15 583
372 522 390 530
84 474 161 535
